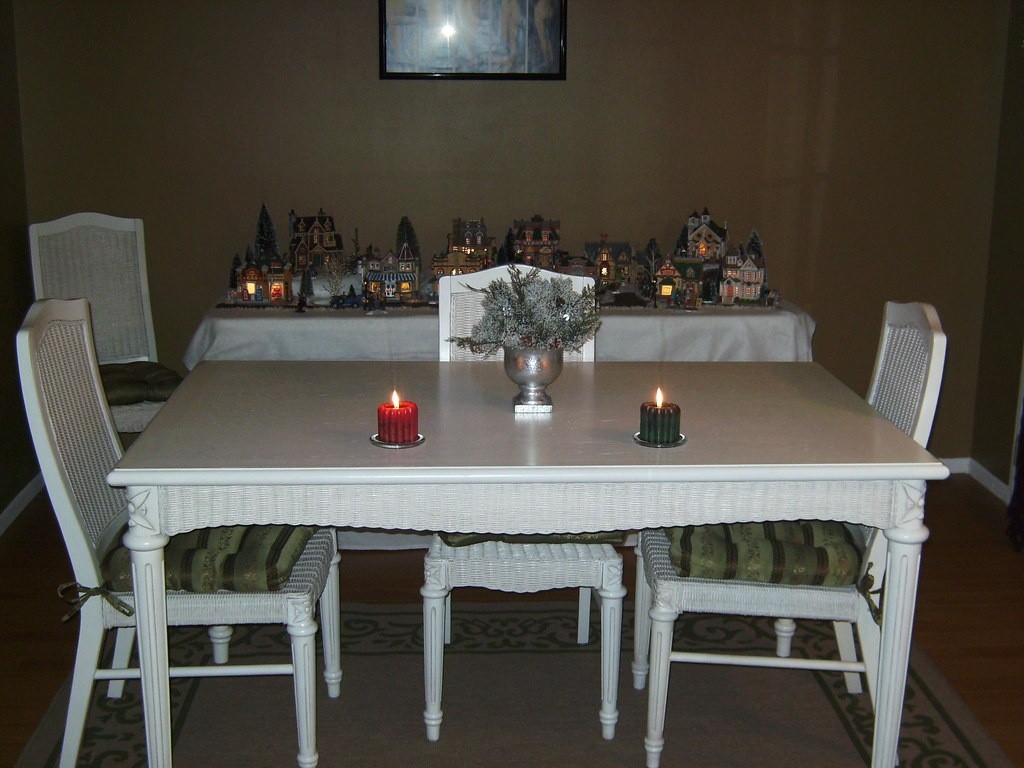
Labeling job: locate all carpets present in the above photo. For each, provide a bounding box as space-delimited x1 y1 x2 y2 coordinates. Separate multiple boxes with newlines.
17 600 1015 768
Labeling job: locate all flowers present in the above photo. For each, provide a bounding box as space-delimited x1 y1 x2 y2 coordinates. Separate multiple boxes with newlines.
444 263 604 354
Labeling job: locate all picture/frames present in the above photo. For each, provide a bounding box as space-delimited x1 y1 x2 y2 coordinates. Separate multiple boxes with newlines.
379 0 567 81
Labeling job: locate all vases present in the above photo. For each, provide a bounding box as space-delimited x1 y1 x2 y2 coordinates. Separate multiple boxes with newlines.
501 346 563 412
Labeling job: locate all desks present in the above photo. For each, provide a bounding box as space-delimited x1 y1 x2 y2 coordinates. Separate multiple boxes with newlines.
104 360 950 768
182 301 816 361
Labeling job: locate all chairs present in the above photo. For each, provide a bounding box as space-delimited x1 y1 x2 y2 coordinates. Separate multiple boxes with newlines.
15 211 948 768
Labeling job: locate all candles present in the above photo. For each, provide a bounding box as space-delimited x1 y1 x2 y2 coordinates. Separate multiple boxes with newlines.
639 387 681 443
377 389 419 444
